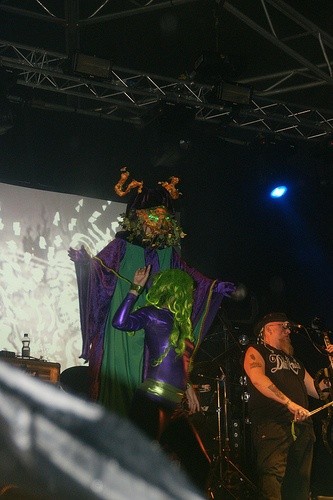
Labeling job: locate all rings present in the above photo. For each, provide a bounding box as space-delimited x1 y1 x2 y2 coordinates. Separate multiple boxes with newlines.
298 410 302 414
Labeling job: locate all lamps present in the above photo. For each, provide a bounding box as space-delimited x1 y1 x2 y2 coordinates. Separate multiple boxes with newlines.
60 49 112 81
207 81 253 109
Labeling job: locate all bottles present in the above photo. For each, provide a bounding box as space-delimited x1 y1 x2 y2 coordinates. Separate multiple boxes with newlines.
22 334 31 359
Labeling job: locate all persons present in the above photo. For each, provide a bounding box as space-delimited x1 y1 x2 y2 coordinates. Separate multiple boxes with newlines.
242 312 333 500
67 164 236 458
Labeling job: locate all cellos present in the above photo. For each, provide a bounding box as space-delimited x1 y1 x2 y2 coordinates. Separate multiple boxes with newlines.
311 310 333 465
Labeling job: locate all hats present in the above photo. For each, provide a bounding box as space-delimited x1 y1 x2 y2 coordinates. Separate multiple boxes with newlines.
260 305 291 325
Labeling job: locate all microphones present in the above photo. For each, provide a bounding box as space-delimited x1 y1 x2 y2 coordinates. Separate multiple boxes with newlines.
283 321 302 328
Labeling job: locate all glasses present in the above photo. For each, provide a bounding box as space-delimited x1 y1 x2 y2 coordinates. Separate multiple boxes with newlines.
267 322 290 330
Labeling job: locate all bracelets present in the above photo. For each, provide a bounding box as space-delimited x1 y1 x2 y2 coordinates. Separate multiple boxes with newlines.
129 283 143 294
284 400 292 406
184 382 192 391
